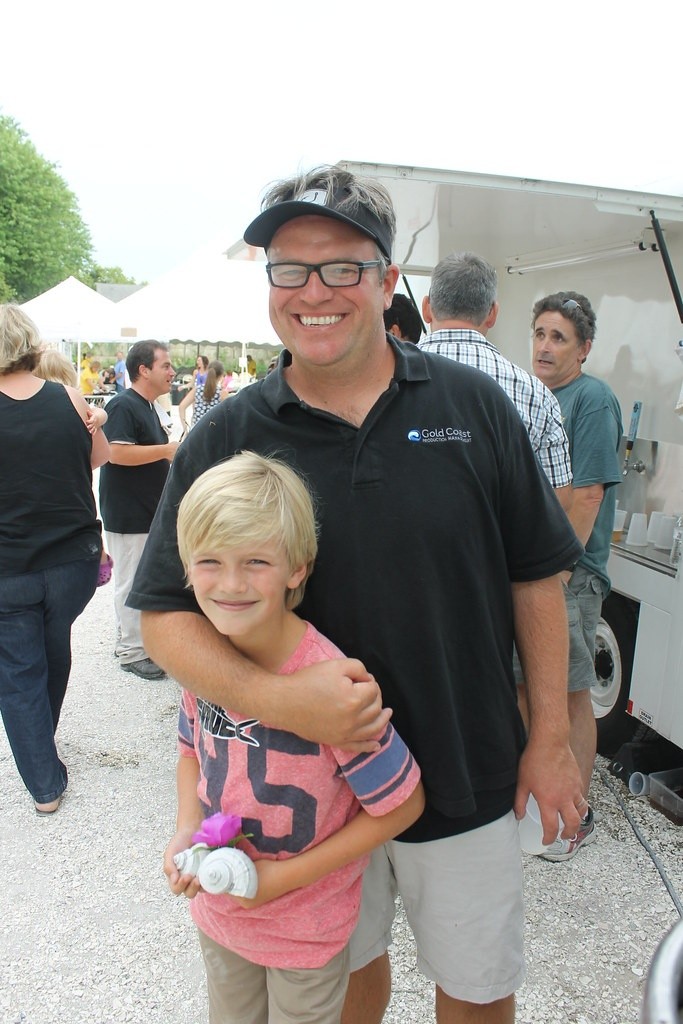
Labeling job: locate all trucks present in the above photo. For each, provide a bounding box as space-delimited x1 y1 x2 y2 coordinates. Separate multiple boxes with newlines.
222 160 683 753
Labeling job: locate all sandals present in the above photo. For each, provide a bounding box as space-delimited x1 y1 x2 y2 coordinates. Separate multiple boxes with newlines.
97 553 112 587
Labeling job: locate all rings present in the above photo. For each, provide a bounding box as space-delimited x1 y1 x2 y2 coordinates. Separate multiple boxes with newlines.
576 798 585 809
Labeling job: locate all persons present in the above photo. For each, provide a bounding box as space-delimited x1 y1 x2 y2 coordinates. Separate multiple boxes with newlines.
179 355 278 435
33 350 113 586
124 168 587 1024
0 304 111 816
384 294 422 346
99 339 182 680
156 393 172 428
532 290 624 861
164 452 425 1024
419 254 574 735
81 352 126 411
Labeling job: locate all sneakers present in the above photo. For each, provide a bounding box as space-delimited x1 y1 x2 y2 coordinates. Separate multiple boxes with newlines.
536 804 598 863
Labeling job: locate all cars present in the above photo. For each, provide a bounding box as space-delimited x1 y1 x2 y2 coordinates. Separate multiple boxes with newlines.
171 367 196 404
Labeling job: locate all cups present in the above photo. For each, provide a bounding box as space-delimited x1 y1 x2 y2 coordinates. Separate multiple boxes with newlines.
612 499 677 549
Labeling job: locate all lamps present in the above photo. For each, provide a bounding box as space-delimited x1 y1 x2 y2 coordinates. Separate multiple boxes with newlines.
504 225 667 276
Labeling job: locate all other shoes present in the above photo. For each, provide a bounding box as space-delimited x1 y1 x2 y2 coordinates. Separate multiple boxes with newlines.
35 800 55 819
122 661 165 679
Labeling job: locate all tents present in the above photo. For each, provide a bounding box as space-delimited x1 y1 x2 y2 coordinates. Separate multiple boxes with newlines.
117 259 284 373
18 276 171 390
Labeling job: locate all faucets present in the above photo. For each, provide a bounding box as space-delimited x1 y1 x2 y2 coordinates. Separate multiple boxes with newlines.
623 400 645 477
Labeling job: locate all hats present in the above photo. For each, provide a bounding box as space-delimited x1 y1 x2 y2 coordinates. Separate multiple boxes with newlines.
243 184 392 267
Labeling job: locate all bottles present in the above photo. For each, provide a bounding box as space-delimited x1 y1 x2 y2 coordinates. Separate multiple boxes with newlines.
669 516 683 566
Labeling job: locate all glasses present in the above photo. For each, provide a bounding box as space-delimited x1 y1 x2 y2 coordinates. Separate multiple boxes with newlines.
265 257 382 288
560 299 586 316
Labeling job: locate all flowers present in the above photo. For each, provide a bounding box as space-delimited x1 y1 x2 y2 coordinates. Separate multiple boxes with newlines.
186 810 257 850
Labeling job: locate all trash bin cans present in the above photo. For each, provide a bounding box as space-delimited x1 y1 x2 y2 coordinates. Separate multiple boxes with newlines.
171 384 186 405
